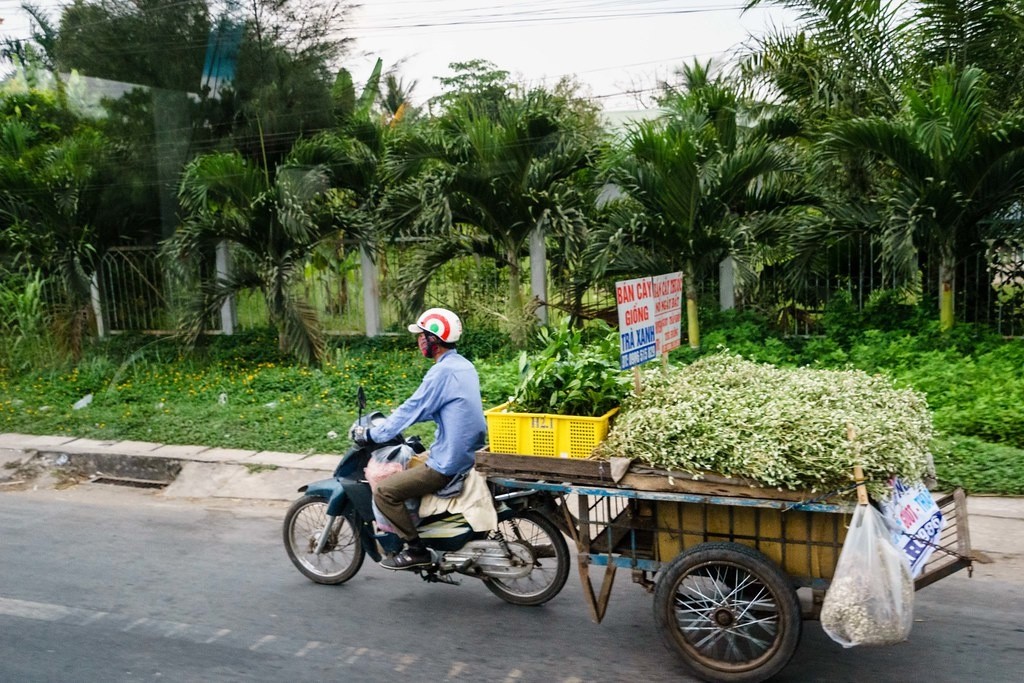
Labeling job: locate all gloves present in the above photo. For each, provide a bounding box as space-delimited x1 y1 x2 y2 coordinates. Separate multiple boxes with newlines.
351 426 369 444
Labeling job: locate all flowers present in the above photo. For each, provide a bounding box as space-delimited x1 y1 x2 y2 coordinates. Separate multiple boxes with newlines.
592 345 939 503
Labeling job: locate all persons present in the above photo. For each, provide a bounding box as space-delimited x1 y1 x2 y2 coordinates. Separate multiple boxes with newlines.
352 308 485 571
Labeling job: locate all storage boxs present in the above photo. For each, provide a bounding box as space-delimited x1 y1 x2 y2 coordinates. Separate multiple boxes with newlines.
483 401 620 459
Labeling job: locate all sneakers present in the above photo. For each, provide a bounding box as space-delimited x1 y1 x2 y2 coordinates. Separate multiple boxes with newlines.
379 548 432 570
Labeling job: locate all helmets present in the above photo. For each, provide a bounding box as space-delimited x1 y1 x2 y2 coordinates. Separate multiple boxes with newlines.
408 308 462 343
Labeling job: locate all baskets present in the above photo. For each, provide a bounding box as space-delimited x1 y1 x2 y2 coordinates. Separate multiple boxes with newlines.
483 400 622 461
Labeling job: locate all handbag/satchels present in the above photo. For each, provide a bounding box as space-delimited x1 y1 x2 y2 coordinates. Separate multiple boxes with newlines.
820 501 915 649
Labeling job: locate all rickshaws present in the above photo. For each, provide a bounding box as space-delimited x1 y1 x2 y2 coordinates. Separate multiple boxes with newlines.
281 385 995 683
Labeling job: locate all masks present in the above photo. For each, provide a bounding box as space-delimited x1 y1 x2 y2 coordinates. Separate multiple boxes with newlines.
418 336 440 357
